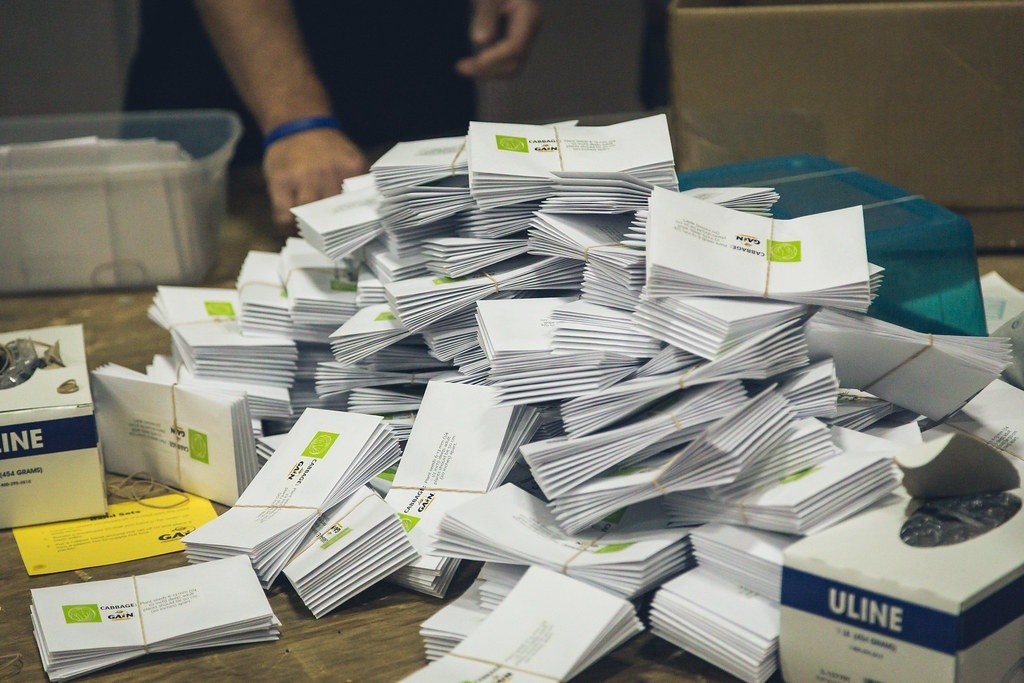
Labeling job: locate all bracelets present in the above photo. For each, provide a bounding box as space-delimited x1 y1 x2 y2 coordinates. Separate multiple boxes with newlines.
261 115 343 149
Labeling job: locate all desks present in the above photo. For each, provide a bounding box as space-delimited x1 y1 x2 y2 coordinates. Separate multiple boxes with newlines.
0 221 1024 683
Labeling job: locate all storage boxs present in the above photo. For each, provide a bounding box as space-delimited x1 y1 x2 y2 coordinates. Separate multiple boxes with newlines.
0 324 109 529
677 152 991 338
779 484 1024 683
0 110 243 294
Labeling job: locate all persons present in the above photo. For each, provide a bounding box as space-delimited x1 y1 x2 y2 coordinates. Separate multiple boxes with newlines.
118 0 536 241
